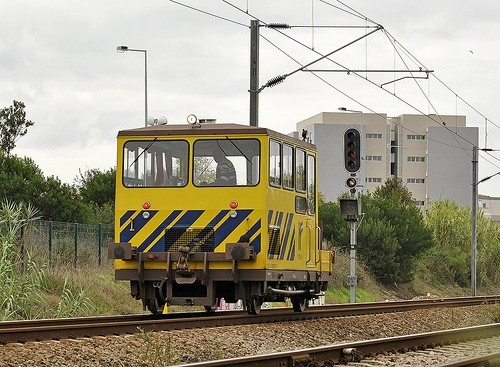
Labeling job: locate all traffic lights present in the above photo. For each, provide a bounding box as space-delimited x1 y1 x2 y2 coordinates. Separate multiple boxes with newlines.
342 128 361 173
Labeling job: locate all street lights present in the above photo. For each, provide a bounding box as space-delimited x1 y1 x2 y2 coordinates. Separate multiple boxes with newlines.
117 44 148 126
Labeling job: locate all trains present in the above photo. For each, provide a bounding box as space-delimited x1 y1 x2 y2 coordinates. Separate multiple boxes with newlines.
114 126 335 313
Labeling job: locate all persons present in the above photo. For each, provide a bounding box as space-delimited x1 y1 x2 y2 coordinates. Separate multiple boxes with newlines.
200 146 237 186
